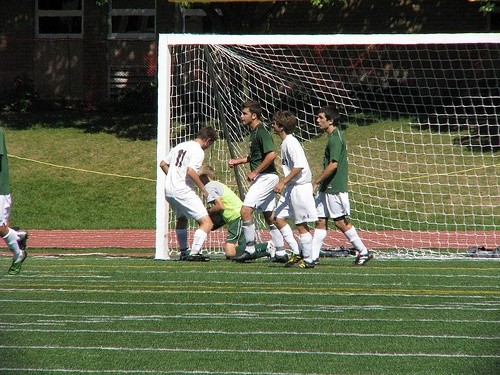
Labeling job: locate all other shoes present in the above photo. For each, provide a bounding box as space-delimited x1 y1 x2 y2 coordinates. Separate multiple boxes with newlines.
8 258 21 275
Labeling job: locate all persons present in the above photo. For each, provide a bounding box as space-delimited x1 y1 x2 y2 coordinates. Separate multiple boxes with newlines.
273 111 319 268
160 126 213 262
199 164 276 260
313 106 372 267
0 128 28 264
228 102 304 268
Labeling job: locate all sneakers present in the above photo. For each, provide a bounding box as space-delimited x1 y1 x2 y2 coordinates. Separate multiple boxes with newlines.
294 259 315 268
265 240 276 259
181 250 209 261
283 250 304 268
313 258 320 265
270 252 288 263
8 231 28 264
355 249 373 265
